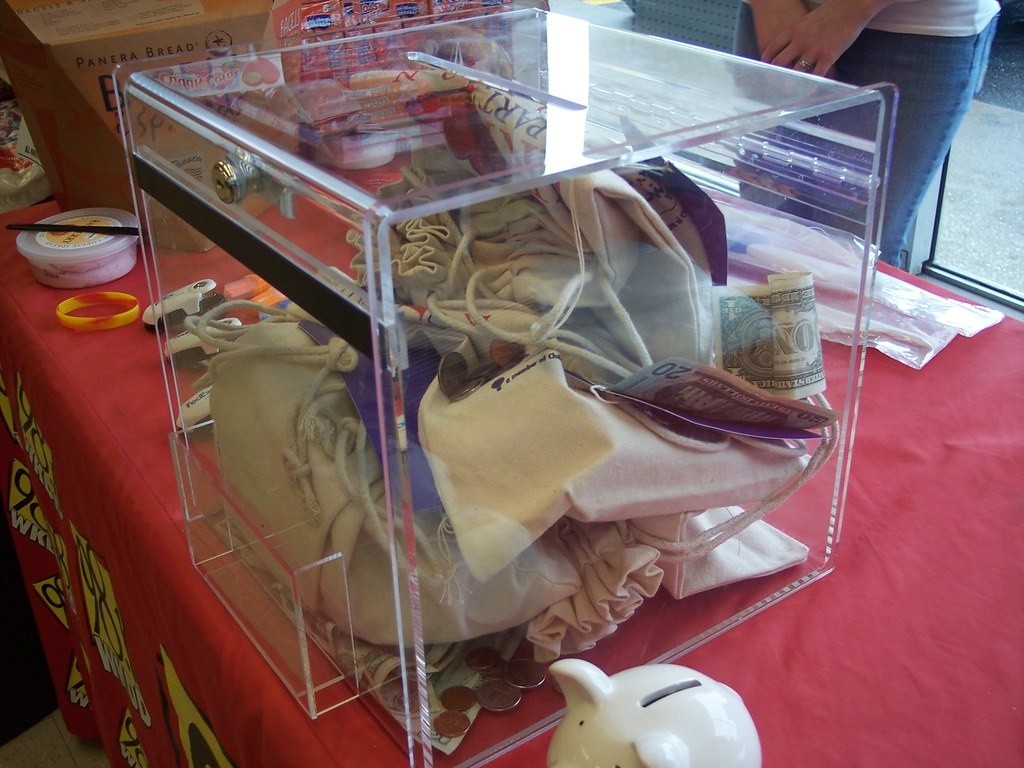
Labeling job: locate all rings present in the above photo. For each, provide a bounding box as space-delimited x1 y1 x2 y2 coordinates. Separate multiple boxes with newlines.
799 59 813 72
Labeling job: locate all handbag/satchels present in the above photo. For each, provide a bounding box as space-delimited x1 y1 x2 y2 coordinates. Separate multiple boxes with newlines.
183 81 839 650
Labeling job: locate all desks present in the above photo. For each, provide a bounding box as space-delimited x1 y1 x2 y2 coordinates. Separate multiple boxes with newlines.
0 204 1023 768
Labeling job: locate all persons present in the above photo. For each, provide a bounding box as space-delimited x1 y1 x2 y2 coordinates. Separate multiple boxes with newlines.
733 0 1000 268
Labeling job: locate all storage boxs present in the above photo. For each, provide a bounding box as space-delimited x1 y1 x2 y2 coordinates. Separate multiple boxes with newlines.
110 5 900 768
0 0 552 220
